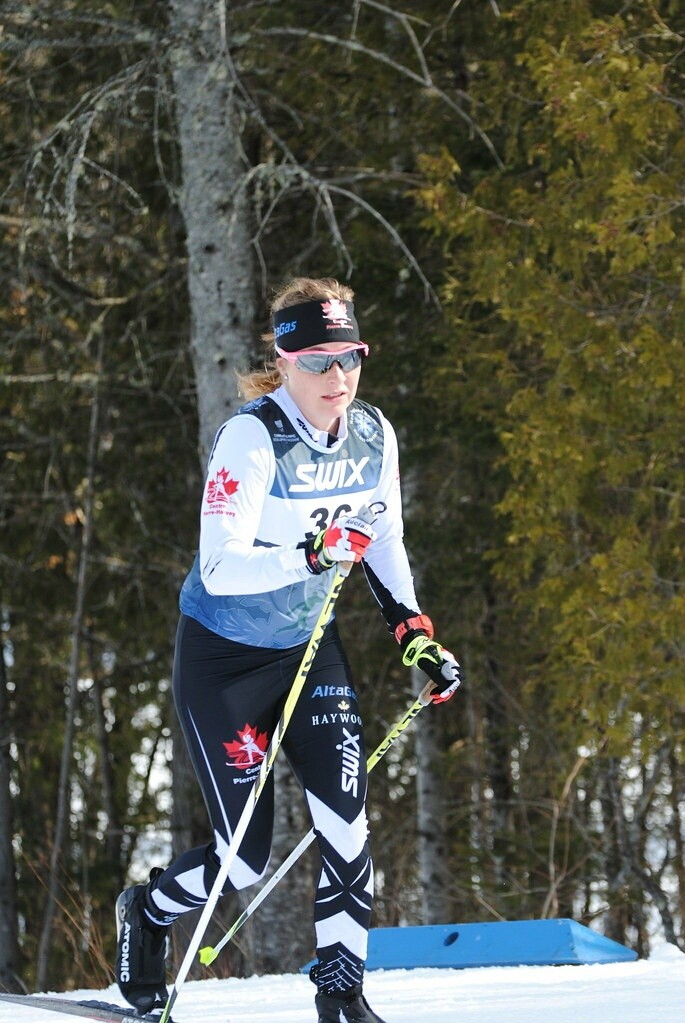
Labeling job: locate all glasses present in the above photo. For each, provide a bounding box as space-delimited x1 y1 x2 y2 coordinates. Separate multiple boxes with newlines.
274 341 369 375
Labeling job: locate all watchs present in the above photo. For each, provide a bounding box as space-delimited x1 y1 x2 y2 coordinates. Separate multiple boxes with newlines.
395 615 434 644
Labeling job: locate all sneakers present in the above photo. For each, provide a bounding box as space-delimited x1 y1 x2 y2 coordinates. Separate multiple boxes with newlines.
115 867 173 1013
315 983 386 1023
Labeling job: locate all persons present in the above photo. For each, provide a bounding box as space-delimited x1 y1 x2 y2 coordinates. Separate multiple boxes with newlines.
115 279 460 1023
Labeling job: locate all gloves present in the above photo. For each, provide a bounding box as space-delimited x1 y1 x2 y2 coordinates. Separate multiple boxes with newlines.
313 515 378 571
402 635 466 705
